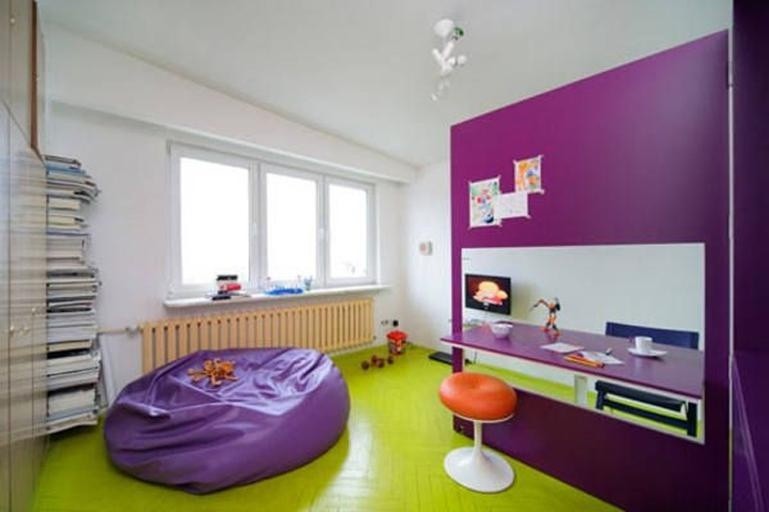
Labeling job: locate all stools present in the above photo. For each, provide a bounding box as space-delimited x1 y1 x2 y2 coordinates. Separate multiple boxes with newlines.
439 371 518 494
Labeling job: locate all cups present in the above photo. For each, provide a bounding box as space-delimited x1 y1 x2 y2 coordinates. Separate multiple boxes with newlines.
635 336 653 352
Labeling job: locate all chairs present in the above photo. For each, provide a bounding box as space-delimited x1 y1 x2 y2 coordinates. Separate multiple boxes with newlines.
594 322 700 436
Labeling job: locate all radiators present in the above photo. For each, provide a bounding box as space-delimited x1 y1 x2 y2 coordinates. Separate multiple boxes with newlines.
139 297 375 377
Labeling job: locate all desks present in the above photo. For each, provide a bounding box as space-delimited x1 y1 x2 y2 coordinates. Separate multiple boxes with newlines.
437 319 705 405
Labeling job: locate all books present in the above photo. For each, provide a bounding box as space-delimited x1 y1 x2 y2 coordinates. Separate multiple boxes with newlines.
46 152 102 434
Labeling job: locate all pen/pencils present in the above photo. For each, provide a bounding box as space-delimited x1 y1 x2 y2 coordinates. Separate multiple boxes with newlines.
563 355 597 369
606 347 613 355
569 353 606 368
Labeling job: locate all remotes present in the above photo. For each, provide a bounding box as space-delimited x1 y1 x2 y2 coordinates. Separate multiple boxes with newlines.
212 295 230 301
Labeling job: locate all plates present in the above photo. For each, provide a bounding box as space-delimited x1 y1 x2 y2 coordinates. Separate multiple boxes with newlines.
627 348 668 357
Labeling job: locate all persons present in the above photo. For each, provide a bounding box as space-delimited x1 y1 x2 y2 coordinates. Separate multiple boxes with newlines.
528 297 560 336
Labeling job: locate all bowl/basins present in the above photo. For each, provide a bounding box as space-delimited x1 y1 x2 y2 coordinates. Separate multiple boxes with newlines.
490 323 513 339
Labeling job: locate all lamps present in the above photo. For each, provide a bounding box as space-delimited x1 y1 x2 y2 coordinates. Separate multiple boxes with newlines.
429 14 472 105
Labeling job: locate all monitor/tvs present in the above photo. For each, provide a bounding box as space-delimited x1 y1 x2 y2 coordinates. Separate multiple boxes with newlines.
465 273 511 315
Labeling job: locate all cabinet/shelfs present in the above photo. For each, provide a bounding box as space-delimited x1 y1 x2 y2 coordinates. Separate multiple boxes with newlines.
0 1 47 162
31 148 51 490
0 98 32 512
